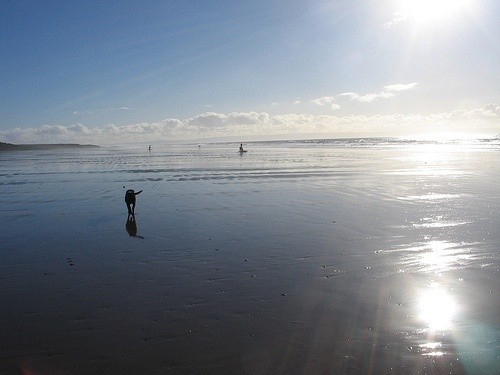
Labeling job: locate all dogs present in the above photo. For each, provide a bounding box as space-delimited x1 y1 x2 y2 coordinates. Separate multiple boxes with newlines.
125 189 143 213
